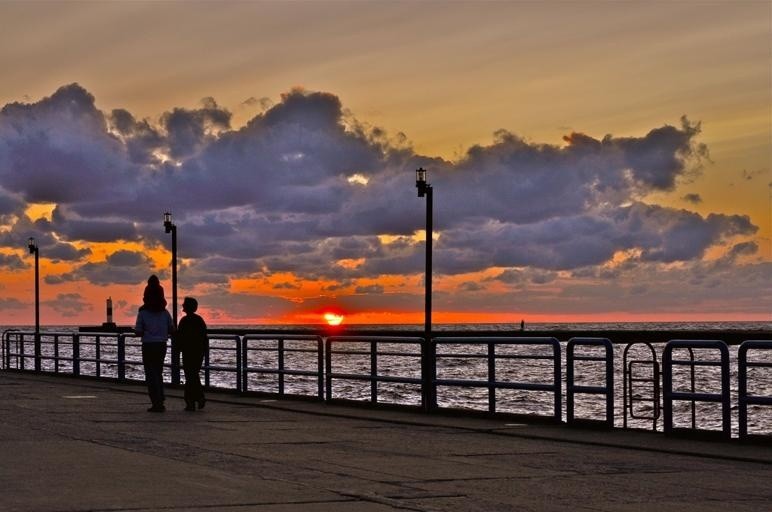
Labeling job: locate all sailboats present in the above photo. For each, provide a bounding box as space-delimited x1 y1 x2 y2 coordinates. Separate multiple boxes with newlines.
79 296 135 332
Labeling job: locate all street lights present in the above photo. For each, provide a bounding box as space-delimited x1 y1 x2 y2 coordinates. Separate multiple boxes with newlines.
416 168 433 331
164 211 177 328
28 236 40 332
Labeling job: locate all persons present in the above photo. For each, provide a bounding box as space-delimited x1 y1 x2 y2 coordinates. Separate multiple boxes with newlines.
133 281 178 412
176 294 209 412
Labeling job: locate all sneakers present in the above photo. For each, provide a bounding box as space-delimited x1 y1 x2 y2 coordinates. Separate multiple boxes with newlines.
146 397 206 413
